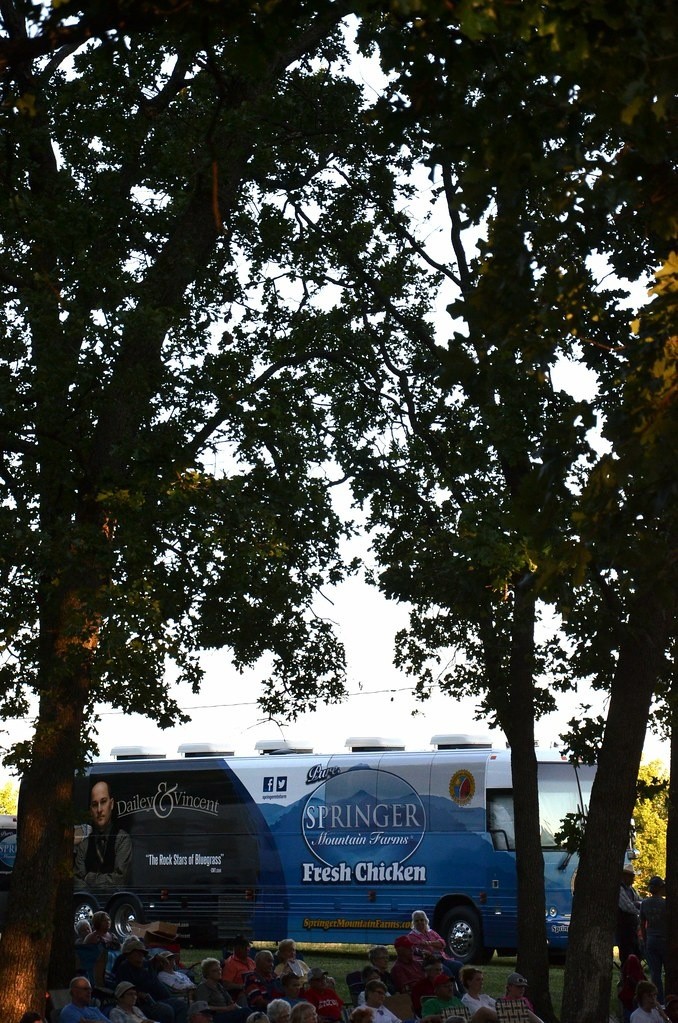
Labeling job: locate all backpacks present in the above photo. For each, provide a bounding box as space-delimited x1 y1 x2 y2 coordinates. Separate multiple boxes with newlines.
614 953 649 1012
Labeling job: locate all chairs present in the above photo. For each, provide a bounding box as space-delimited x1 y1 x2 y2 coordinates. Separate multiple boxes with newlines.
43 937 649 1023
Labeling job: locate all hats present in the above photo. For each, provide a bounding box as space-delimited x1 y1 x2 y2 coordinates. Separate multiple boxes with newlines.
432 972 456 986
233 935 253 946
394 936 414 949
156 950 179 967
307 967 329 984
665 994 678 1010
186 1000 216 1016
508 972 529 987
126 941 149 955
114 981 136 999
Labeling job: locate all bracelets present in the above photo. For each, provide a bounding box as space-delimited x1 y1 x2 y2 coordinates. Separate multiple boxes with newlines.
425 941 431 946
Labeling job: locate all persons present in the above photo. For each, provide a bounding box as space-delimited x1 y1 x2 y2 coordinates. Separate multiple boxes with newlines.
73 779 135 895
615 862 678 1022
19 911 534 1023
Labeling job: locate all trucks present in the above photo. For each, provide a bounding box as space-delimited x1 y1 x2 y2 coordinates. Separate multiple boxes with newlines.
72 733 641 967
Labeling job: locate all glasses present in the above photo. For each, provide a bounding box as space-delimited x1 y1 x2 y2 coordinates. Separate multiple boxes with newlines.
253 1012 265 1023
516 977 528 983
440 984 453 988
374 990 385 996
430 967 441 972
209 967 222 972
314 975 326 983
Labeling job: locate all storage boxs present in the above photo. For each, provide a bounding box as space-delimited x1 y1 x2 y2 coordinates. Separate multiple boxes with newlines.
131 920 178 944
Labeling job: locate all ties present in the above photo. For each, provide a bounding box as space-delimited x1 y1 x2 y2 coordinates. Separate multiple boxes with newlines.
96 833 108 858
